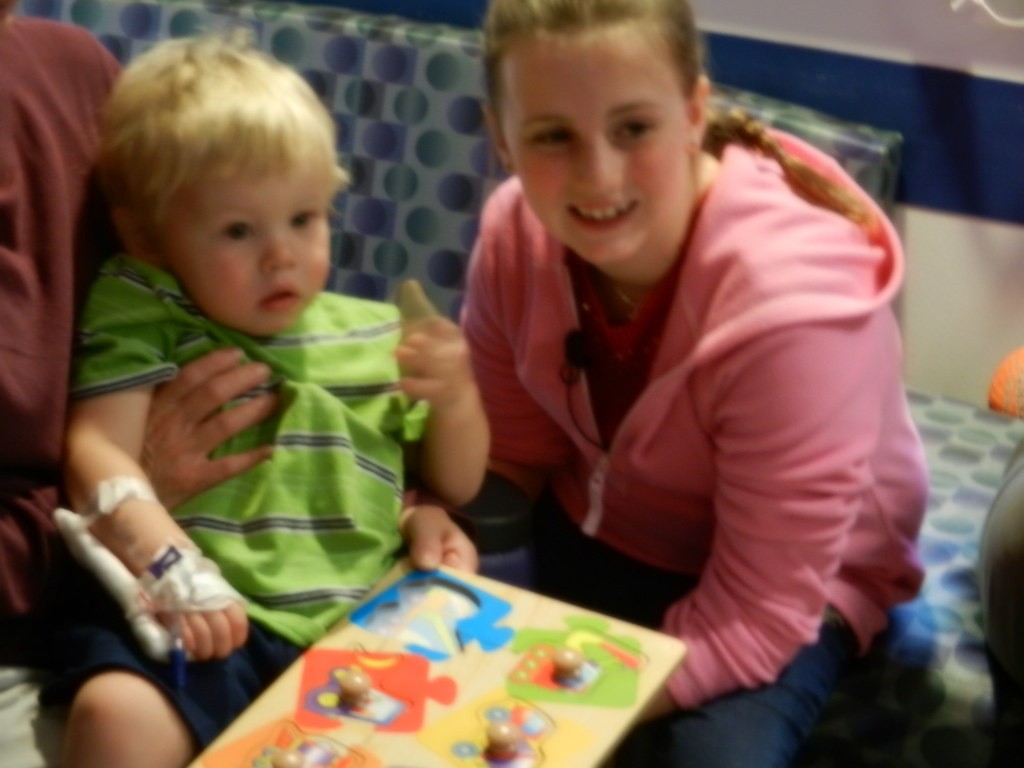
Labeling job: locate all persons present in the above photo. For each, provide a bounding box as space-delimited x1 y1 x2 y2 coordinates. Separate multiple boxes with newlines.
455 1 927 768
67 29 491 768
0 0 481 714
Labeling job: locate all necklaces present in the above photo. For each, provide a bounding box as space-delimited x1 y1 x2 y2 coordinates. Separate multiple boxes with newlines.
613 285 637 310
